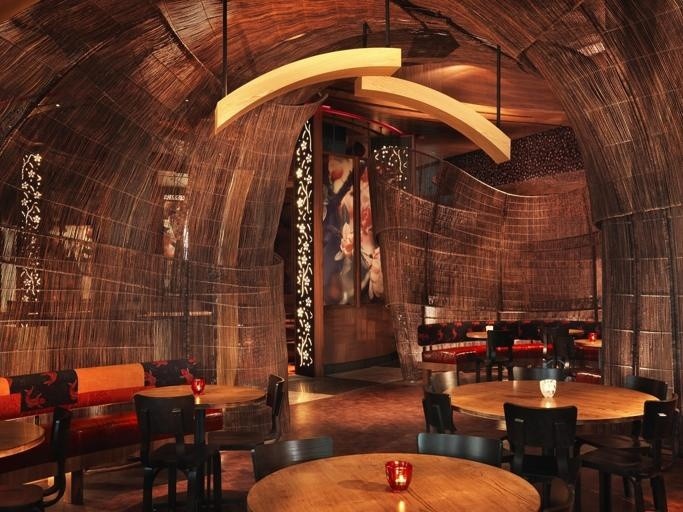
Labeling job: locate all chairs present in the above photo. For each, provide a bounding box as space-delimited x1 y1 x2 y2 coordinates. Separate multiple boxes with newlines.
453 328 577 373
133 372 337 512
413 365 675 512
0 404 72 511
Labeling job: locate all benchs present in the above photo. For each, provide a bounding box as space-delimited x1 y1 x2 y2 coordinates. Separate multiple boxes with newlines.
0 358 227 512
416 317 603 392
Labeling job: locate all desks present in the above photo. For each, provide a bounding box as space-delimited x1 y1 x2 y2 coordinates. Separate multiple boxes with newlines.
249 450 543 512
0 417 47 465
